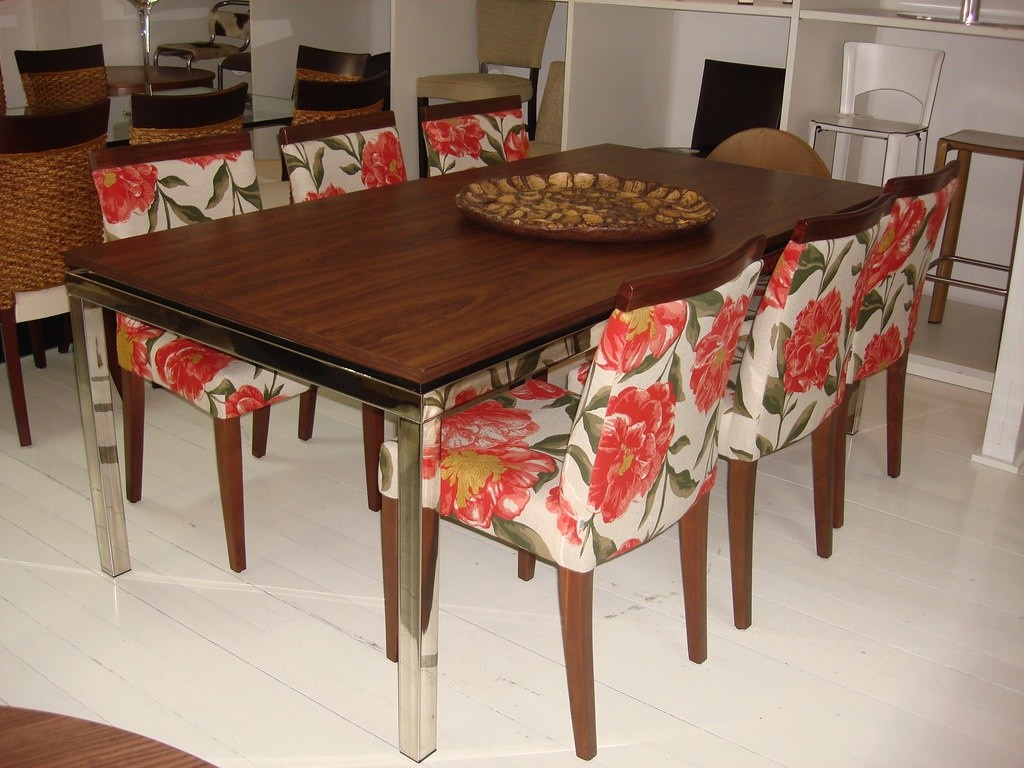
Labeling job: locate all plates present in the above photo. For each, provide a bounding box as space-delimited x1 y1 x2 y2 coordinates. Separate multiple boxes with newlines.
456 173 718 241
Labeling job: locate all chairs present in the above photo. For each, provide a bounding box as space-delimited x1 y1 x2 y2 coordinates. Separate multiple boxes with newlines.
0 39 965 761
416 0 557 176
153 0 251 68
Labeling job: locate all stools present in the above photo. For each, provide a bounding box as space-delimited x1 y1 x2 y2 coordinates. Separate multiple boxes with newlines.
927 128 1024 376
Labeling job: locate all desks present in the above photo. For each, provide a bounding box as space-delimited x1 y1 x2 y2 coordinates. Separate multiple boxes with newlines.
0 66 217 150
59 142 891 672
